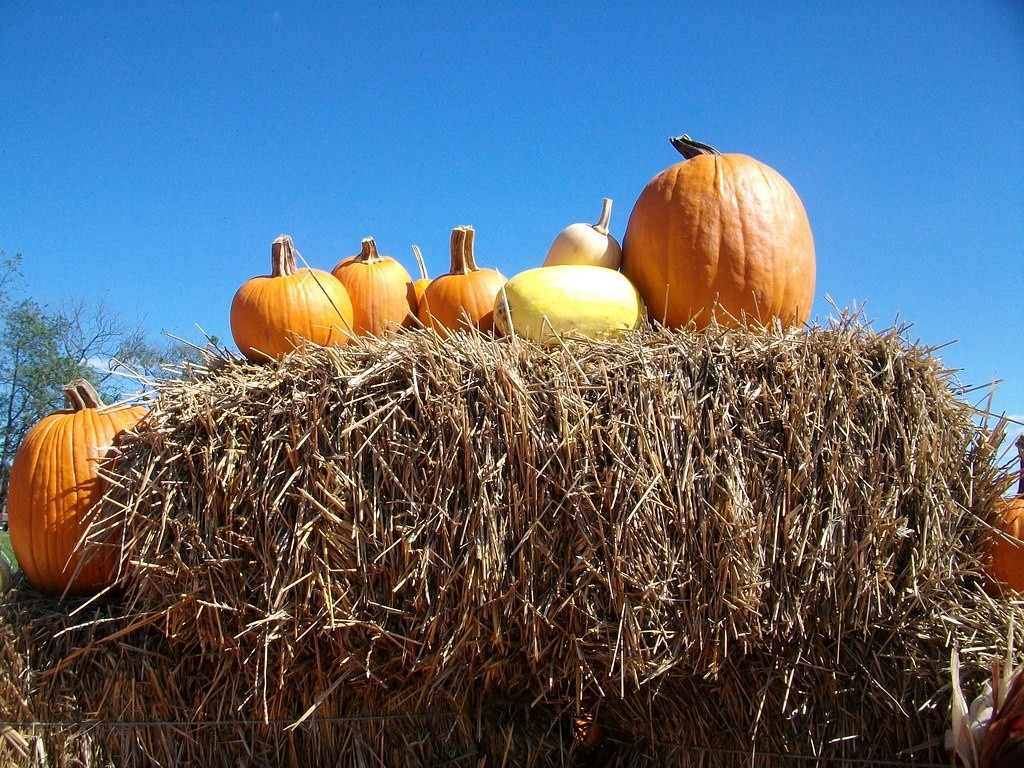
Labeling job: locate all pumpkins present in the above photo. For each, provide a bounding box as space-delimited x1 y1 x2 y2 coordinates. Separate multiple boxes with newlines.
976 433 1024 602
6 378 152 598
229 134 816 360
